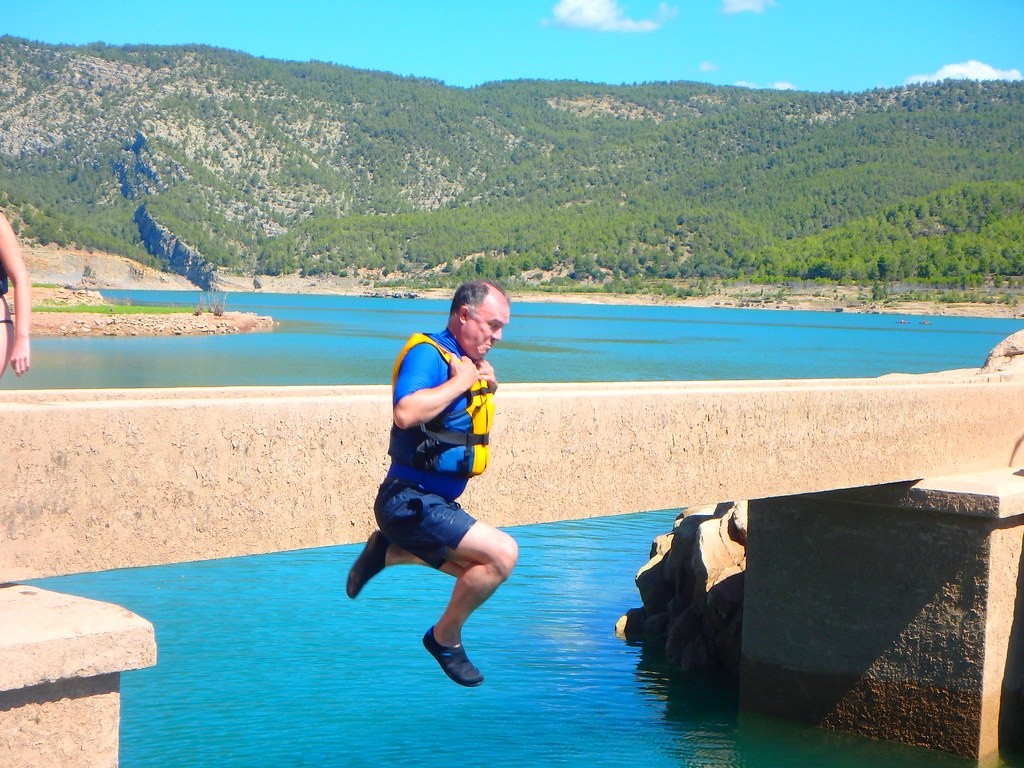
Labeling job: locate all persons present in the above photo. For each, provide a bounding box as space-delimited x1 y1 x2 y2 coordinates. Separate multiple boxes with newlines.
0 209 31 377
347 282 517 686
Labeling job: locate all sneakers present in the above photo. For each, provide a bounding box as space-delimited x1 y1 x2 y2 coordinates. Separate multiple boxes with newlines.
423 626 484 686
347 530 391 599
